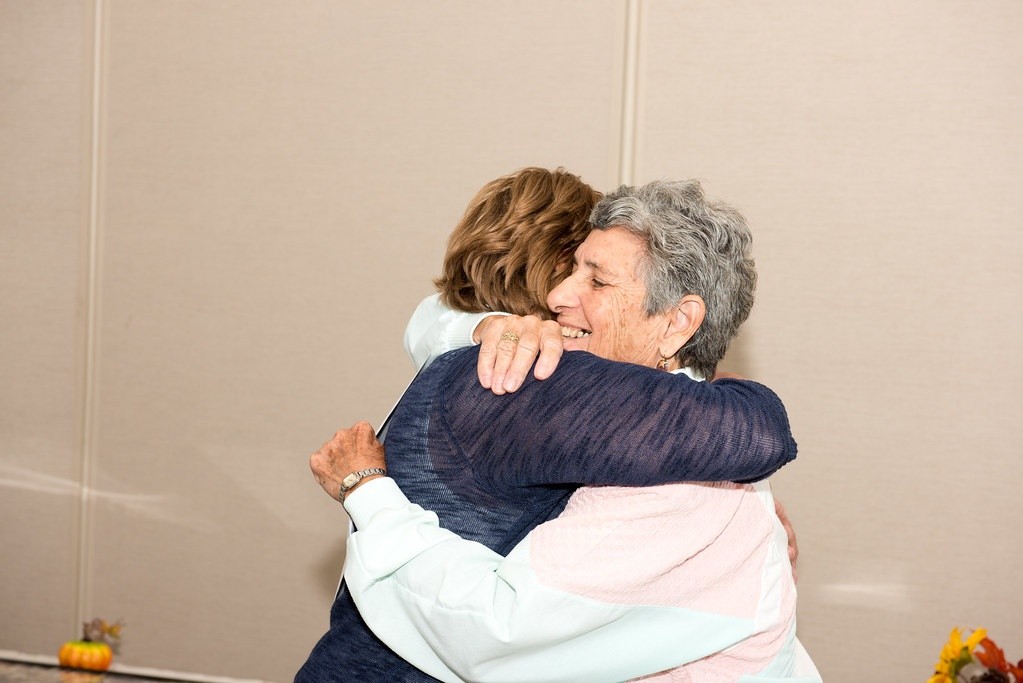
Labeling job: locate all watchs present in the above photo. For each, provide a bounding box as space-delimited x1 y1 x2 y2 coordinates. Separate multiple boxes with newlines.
337 468 386 505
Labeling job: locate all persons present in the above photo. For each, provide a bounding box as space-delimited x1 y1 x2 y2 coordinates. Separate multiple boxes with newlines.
294 168 797 682
309 179 825 683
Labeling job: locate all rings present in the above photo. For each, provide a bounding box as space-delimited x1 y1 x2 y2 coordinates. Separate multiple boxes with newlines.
497 331 519 343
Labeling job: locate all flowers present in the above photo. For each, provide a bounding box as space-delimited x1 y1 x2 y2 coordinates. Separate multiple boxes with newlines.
924 624 1023 683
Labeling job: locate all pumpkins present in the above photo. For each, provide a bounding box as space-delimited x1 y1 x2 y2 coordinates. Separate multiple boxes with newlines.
57 626 112 670
